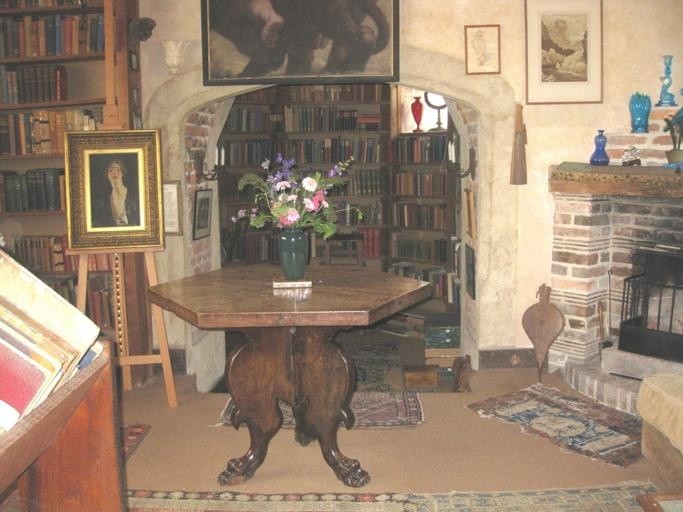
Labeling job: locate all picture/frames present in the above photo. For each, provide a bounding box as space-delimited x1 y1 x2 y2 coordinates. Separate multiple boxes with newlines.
194 189 212 240
464 188 476 240
524 0 604 105
464 25 500 74
163 181 182 236
200 0 400 85
64 130 163 249
465 245 476 300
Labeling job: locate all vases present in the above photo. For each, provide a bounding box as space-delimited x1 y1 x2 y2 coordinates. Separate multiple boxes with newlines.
412 97 423 133
278 230 307 280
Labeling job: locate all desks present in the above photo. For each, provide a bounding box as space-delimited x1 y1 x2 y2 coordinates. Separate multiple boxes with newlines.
149 264 432 486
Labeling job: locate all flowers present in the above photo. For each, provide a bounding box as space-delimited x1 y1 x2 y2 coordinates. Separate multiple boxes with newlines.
233 152 363 240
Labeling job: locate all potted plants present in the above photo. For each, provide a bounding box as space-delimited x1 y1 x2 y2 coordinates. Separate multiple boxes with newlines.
664 115 683 164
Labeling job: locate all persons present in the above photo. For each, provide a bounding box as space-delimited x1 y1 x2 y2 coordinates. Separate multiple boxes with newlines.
95 154 139 226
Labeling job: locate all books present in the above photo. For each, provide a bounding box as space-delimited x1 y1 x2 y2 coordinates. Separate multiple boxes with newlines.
392 135 460 306
215 82 390 272
1 107 102 212
1 1 105 106
272 275 313 288
1 233 113 431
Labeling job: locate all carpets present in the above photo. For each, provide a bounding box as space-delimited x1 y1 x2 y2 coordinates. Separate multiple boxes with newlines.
123 425 658 512
466 382 646 469
213 391 424 427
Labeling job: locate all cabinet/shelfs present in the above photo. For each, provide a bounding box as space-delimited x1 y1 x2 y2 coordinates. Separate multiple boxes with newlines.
216 85 458 306
0 0 150 386
